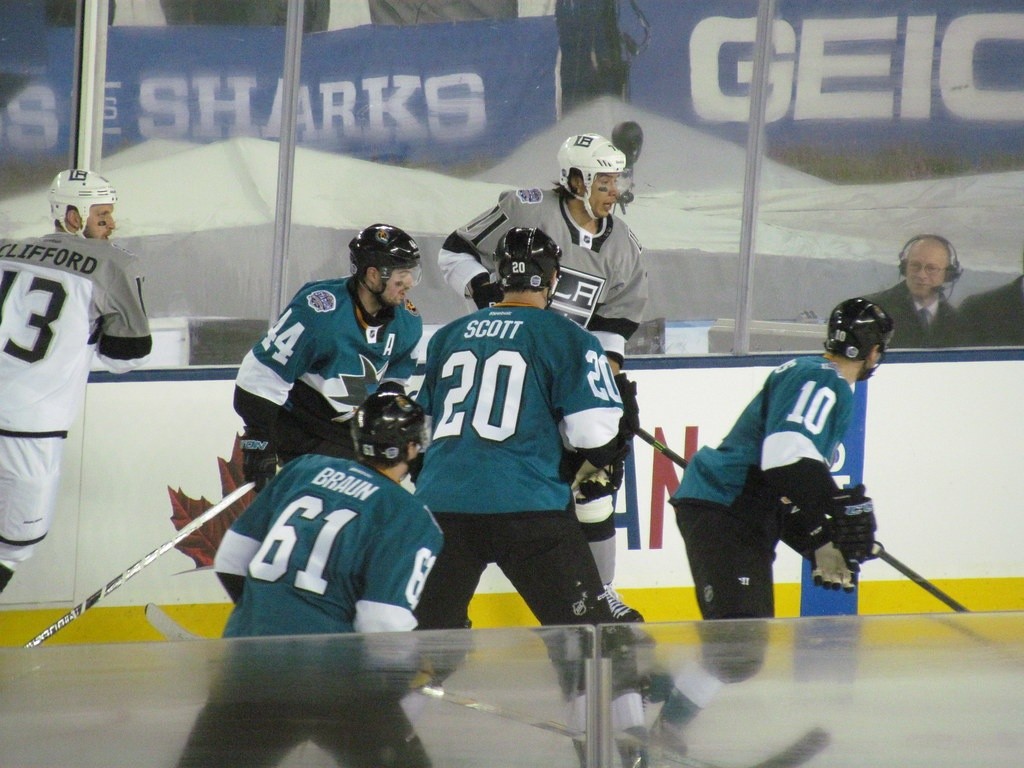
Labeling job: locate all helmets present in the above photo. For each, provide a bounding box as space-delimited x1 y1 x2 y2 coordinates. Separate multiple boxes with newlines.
558 132 627 203
349 391 428 467
347 223 425 296
47 169 120 240
824 296 892 363
492 226 563 290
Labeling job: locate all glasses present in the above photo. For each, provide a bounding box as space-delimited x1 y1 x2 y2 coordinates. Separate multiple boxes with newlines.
903 259 945 278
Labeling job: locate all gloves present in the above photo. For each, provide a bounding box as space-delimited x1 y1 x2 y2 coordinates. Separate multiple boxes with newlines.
615 372 641 441
575 459 625 504
239 426 280 492
829 482 879 575
806 524 862 594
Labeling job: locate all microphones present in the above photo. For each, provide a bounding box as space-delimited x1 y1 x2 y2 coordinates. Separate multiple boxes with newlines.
932 269 964 293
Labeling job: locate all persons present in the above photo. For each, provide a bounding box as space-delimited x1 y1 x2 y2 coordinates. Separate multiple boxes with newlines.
408 226 649 768
177 390 446 768
859 234 1024 348
650 297 897 755
233 224 431 491
0 170 152 595
436 133 650 624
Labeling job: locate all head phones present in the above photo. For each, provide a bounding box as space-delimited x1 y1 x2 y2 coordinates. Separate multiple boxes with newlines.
898 234 959 282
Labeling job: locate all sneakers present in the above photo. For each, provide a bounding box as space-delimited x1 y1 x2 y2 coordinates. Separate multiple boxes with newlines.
650 696 701 759
601 581 646 623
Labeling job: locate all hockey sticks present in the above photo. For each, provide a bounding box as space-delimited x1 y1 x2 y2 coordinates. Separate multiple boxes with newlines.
24 480 255 649
871 544 970 613
416 683 829 768
638 426 686 467
